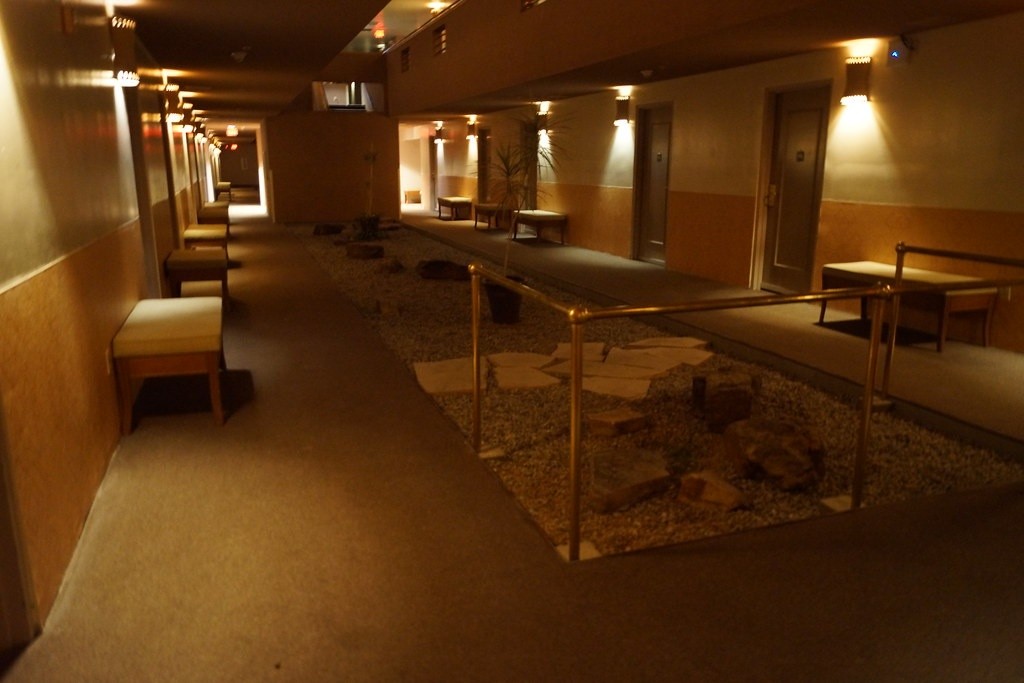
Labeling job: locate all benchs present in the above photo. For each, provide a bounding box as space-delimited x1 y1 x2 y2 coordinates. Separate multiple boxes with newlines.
400 186 567 249
820 261 999 350
110 182 231 432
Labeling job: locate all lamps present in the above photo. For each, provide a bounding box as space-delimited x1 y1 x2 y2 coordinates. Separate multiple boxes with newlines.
164 83 222 158
465 120 478 141
840 56 873 108
108 15 141 88
613 95 630 128
434 127 442 144
536 110 549 136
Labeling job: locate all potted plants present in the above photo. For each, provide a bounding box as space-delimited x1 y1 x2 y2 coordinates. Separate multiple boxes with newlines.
358 145 384 234
460 104 575 329
